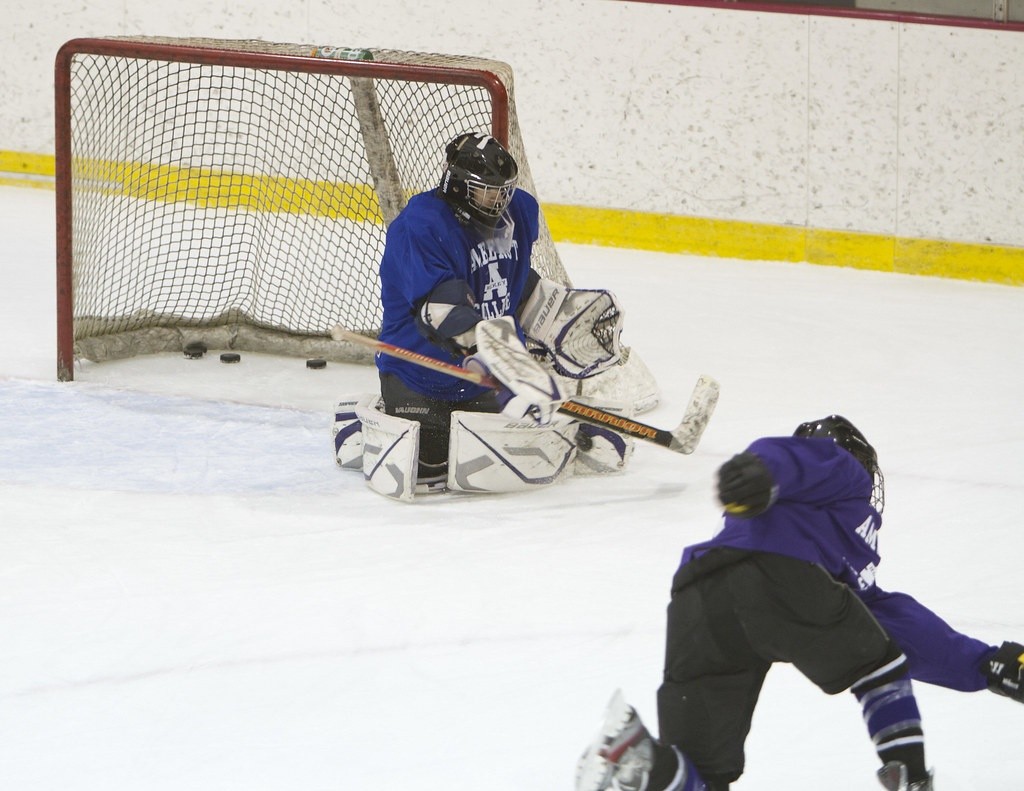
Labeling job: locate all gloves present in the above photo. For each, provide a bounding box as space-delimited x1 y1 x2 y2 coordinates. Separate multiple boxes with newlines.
715 450 780 521
978 639 1024 703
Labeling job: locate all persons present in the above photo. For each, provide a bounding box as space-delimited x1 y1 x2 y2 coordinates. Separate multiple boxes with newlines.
335 133 661 505
574 414 1024 791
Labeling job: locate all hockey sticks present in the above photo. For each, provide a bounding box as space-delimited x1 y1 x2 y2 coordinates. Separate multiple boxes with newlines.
327 323 724 456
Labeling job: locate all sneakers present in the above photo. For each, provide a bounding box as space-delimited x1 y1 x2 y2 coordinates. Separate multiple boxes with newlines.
876 760 935 791
572 702 687 791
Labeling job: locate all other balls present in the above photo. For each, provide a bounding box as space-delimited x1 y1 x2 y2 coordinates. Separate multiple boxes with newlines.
183 346 208 360
306 359 326 370
219 353 241 364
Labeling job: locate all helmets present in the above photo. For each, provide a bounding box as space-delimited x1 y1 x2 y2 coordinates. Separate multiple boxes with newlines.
437 131 519 238
791 414 885 516
